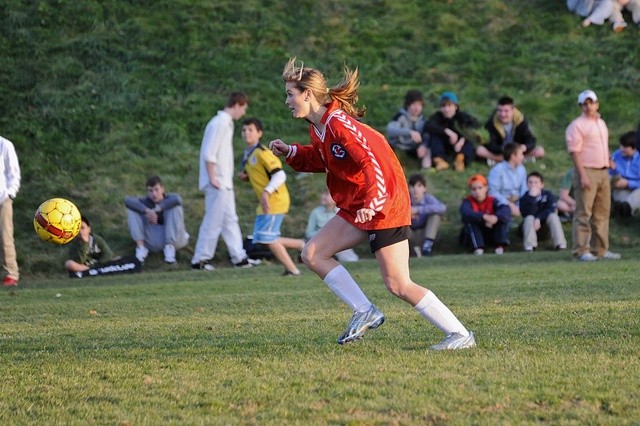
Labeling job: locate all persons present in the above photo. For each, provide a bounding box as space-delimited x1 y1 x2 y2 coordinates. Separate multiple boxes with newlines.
295 188 358 263
124 176 190 264
565 0 631 27
423 91 478 173
407 174 447 258
475 96 545 167
458 174 512 256
609 0 640 32
557 166 576 222
385 89 433 170
519 171 567 251
486 142 529 225
608 131 640 218
0 136 21 287
60 216 122 279
237 118 309 277
189 91 262 272
565 90 621 262
269 55 477 351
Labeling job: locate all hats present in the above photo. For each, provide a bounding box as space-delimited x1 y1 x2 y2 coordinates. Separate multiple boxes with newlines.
468 174 487 185
440 91 458 103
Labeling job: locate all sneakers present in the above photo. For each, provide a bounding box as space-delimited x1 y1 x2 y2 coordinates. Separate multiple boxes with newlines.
598 252 621 259
575 253 599 261
455 153 465 170
431 331 477 349
577 89 597 103
434 157 449 169
163 244 176 263
336 304 385 344
135 246 149 262
192 260 215 271
422 238 433 255
613 20 627 31
2 277 17 286
232 257 261 268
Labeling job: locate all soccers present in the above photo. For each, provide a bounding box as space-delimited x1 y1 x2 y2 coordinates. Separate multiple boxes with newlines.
34 198 82 245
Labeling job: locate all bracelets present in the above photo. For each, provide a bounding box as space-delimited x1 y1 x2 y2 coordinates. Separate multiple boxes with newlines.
9 195 15 201
